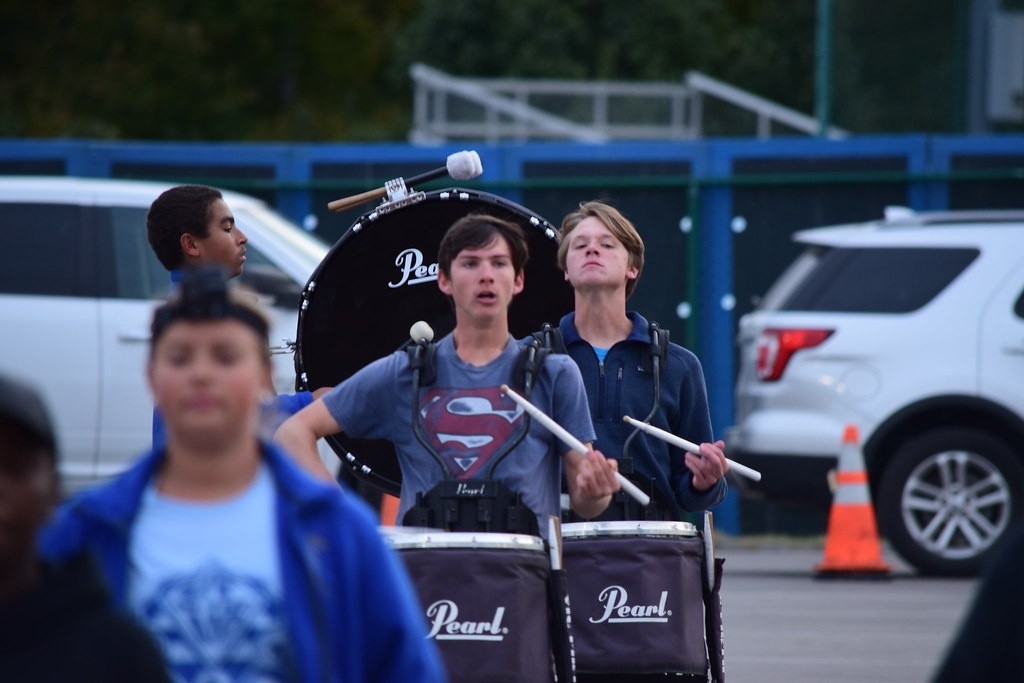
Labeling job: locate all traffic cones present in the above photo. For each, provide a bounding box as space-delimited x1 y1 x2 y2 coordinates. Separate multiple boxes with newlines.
806 424 898 580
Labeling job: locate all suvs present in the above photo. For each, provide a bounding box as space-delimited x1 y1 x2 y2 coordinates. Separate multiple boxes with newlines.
717 203 1023 577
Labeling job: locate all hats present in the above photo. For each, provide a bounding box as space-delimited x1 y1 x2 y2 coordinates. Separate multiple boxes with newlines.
0 378 55 462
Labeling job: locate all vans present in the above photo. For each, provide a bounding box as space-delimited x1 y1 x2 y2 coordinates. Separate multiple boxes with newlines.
0 178 367 509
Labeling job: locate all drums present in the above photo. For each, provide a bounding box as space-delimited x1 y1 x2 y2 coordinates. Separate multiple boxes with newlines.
377 530 553 683
562 519 707 683
293 182 574 499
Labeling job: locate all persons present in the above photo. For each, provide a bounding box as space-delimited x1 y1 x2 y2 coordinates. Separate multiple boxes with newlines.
522 200 728 519
0 185 621 683
